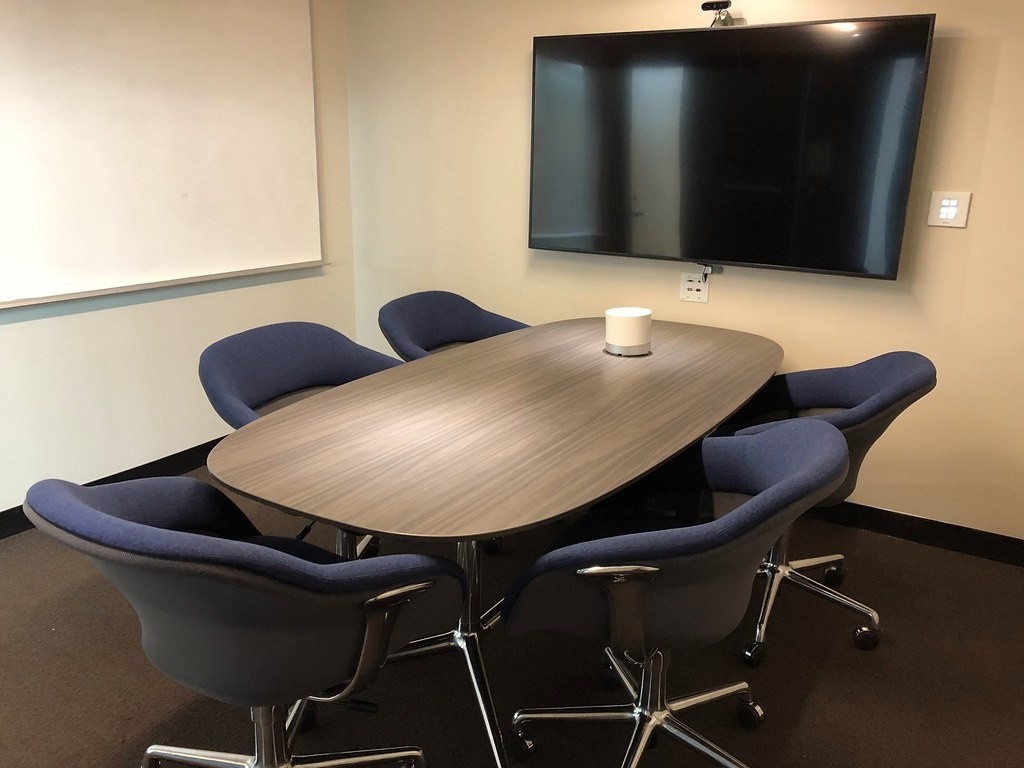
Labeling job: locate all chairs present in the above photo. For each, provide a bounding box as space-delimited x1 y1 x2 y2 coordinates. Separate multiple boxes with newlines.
379 291 528 556
198 321 402 561
716 350 936 668
510 417 849 768
24 479 438 768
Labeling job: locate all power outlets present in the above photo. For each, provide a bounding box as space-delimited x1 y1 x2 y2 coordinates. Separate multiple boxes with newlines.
678 272 708 303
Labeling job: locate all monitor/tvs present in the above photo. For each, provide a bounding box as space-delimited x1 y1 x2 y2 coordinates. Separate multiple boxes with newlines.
527 13 938 280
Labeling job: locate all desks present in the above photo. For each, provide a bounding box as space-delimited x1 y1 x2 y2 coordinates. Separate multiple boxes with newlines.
206 316 783 768
